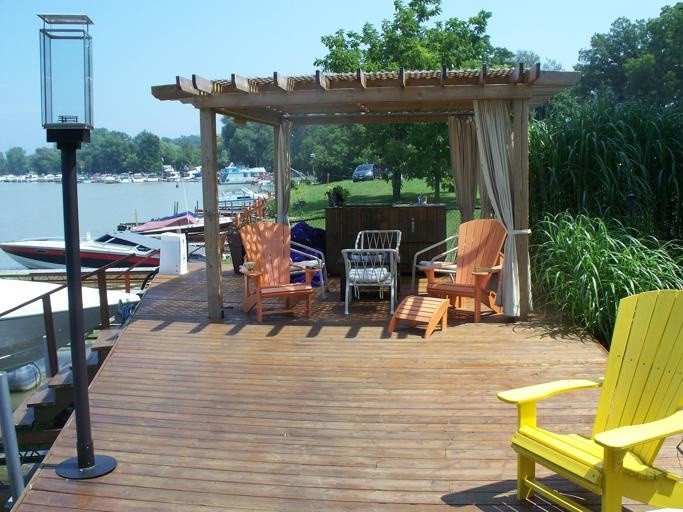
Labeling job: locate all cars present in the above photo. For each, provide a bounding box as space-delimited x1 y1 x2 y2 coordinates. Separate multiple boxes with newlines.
352 163 379 182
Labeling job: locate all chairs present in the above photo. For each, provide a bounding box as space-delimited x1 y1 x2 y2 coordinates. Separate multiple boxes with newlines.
494 290 682 512
289 239 327 299
341 230 399 317
239 223 327 324
386 218 503 339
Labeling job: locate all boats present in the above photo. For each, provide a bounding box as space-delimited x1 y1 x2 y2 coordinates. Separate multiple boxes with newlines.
0 224 230 271
1 276 143 373
0 165 276 187
117 185 272 243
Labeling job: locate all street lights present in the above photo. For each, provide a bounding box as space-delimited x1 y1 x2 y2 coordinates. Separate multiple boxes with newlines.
35 12 118 483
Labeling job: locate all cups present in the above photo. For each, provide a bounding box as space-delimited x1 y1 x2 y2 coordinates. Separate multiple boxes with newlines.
419 195 428 204
244 262 257 274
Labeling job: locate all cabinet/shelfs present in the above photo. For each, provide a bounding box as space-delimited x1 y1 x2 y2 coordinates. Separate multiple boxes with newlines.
323 206 446 277
